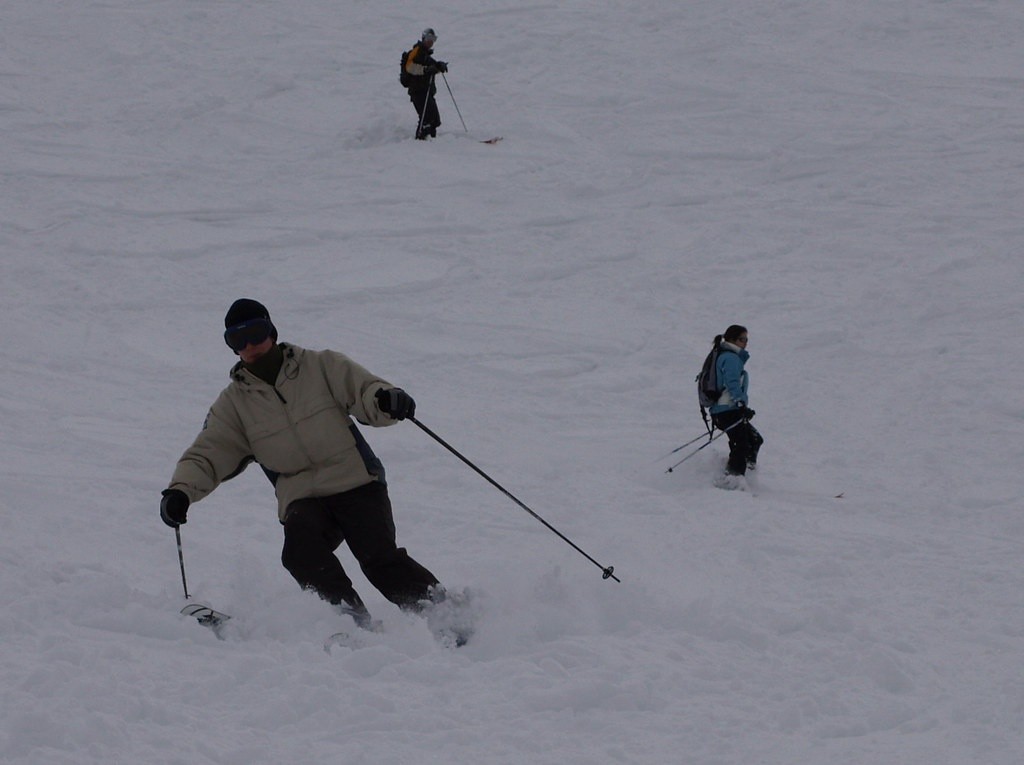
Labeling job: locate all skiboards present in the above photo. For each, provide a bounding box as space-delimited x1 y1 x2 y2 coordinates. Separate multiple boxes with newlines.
177 601 486 648
458 138 500 146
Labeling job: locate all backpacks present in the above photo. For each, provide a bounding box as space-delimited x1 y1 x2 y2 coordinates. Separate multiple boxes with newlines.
400 43 427 88
698 343 743 407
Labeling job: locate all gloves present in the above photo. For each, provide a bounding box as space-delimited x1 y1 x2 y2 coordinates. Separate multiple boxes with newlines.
160 488 190 528
425 65 438 75
378 388 415 421
435 62 448 73
740 402 754 424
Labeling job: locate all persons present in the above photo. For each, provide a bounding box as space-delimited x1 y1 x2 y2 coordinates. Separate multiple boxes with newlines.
161 297 473 646
400 29 448 140
695 325 763 488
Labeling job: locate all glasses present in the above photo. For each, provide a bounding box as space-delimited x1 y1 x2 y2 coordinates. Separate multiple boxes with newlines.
229 322 269 351
735 337 748 343
424 33 436 41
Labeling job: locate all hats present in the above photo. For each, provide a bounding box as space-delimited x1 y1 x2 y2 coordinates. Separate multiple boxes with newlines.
224 299 278 354
422 28 435 41
